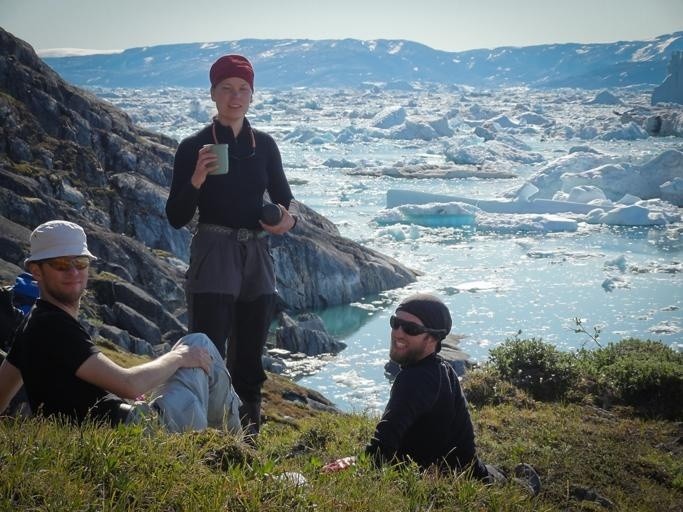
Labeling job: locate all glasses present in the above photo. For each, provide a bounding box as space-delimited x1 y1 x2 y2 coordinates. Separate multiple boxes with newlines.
46 256 89 271
390 315 446 337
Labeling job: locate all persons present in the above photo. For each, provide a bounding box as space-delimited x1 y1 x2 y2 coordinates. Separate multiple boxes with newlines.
0 220 244 437
364 294 505 488
166 55 301 448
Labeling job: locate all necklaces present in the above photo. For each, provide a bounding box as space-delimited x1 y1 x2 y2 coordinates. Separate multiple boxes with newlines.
212 123 256 161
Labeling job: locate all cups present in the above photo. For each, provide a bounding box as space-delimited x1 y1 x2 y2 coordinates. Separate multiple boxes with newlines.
203 143 228 176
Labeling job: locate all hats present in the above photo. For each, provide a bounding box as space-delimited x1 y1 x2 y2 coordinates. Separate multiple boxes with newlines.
395 292 453 341
209 54 256 90
23 219 97 271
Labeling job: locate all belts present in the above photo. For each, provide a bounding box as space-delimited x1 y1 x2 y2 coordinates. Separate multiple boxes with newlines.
113 403 133 427
201 222 268 241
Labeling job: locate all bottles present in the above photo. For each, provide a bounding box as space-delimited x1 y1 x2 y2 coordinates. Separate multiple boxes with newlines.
259 203 281 225
